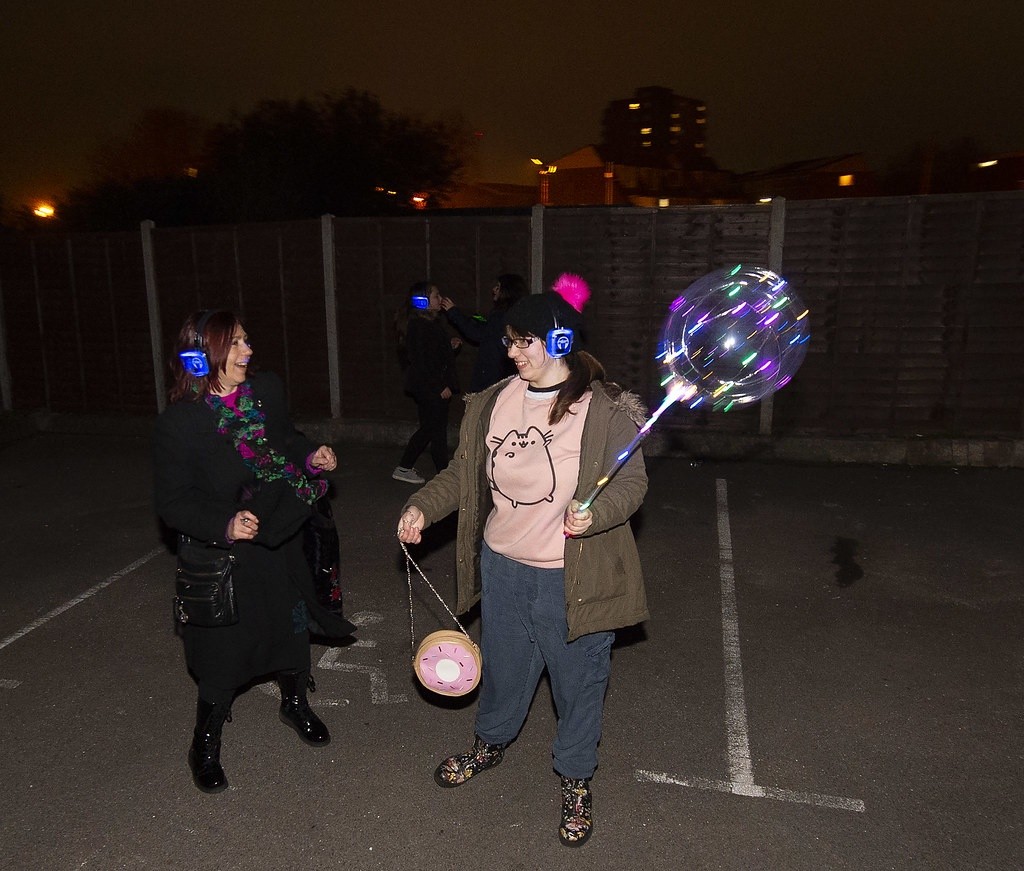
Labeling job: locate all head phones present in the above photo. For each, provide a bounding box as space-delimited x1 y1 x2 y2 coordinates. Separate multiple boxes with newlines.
534 293 574 359
179 308 228 378
410 284 429 310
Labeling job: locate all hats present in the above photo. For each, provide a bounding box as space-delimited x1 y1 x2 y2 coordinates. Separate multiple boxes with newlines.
503 274 591 354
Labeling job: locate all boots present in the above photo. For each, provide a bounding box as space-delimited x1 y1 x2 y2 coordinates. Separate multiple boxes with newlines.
275 667 330 747
188 697 234 794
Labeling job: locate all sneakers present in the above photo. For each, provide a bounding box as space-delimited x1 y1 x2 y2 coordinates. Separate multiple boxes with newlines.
392 465 425 484
559 775 593 848
435 730 508 788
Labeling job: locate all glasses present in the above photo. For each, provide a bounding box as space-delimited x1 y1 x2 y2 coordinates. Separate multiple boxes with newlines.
501 335 540 349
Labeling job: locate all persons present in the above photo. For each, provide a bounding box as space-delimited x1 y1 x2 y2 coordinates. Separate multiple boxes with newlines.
392 273 653 850
153 307 357 795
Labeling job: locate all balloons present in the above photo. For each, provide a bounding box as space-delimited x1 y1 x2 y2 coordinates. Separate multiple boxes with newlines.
660 265 812 411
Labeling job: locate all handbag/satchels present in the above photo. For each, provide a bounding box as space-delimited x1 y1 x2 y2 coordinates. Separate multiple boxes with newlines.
413 630 483 697
173 560 239 627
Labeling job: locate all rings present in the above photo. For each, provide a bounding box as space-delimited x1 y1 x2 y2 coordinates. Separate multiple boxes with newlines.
240 518 250 525
